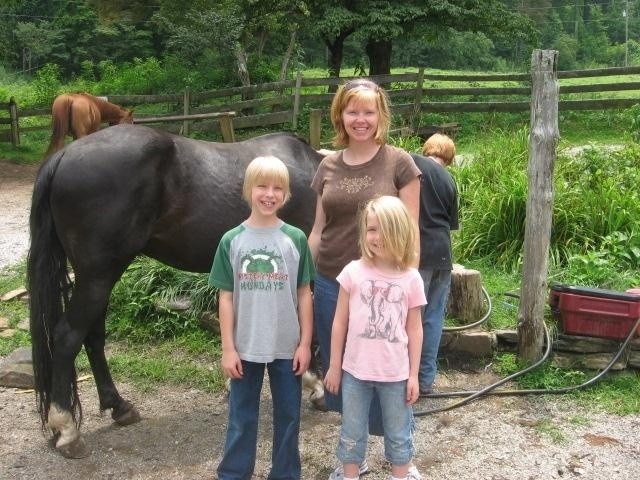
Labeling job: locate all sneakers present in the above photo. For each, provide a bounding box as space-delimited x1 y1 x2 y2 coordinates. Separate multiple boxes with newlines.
328 459 368 480
391 464 423 480
419 388 433 394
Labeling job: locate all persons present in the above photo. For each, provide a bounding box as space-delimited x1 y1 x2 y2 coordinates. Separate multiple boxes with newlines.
322 195 430 480
315 132 461 393
205 154 320 480
307 78 424 480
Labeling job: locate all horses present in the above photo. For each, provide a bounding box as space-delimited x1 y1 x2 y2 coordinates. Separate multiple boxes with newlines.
24 121 329 461
45 91 134 160
